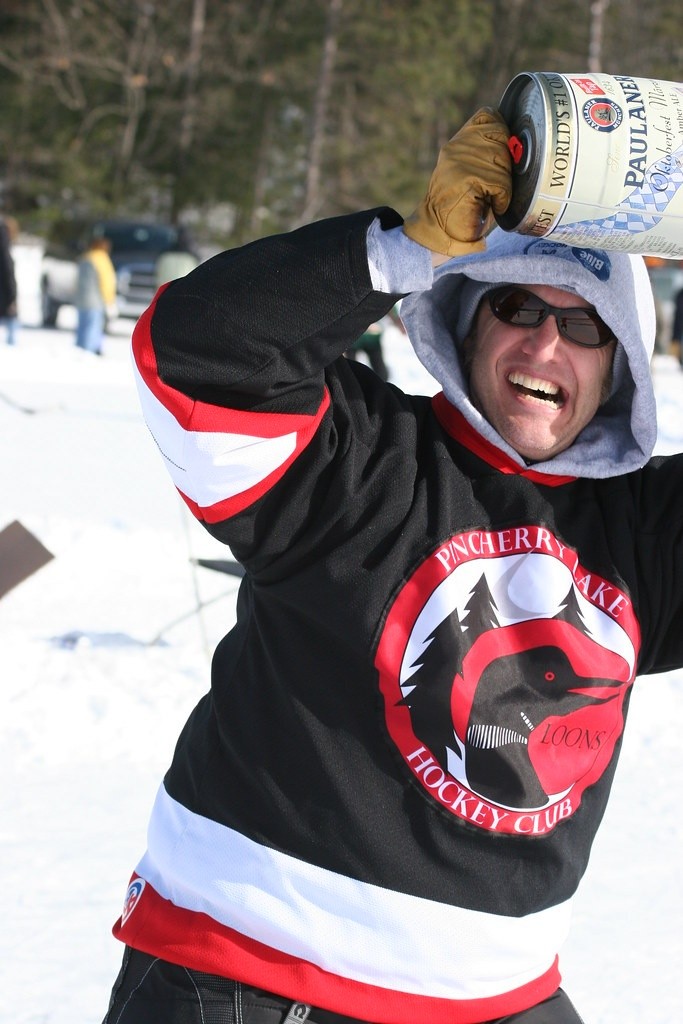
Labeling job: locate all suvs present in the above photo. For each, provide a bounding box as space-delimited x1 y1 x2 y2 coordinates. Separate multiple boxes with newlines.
38 218 201 328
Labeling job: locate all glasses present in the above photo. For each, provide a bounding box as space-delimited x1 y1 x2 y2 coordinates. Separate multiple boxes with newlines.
478 282 618 349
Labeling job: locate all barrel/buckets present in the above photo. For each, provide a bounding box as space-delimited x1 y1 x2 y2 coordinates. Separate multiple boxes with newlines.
493 72 683 258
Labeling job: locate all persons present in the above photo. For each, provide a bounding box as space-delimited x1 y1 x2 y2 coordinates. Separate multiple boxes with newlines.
153 220 201 293
102 105 683 1024
74 237 118 355
344 304 407 383
0 214 20 345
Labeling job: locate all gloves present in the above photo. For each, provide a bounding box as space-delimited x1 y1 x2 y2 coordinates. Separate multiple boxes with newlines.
403 106 513 257
